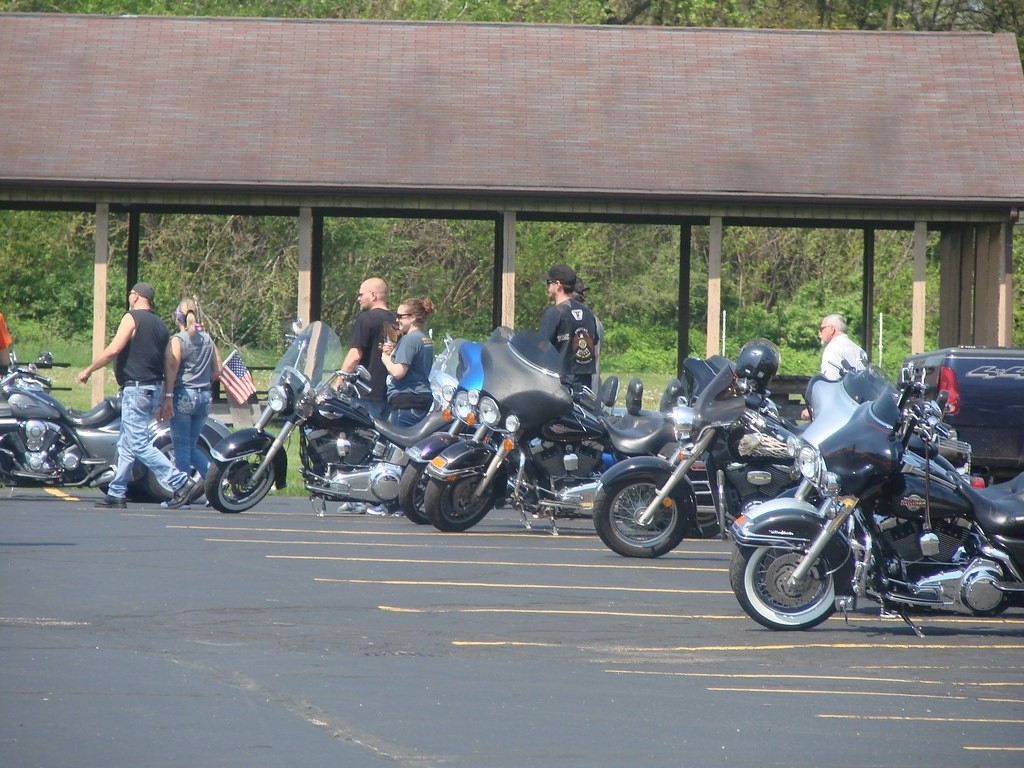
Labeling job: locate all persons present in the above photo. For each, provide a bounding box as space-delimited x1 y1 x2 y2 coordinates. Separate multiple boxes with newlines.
317 277 407 515
366 296 437 517
800 313 869 422
530 264 604 519
0 312 13 368
159 297 224 511
76 281 201 510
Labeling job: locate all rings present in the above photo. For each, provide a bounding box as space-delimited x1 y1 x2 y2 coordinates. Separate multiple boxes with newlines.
160 417 164 421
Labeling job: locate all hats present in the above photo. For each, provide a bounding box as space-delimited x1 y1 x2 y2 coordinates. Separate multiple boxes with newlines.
133 282 154 301
541 264 576 285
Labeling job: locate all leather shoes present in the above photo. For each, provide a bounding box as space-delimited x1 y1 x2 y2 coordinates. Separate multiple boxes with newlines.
168 477 201 509
94 495 127 509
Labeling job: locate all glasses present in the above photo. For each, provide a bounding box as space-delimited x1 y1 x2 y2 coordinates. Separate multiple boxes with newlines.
396 314 412 319
820 326 833 332
547 280 556 285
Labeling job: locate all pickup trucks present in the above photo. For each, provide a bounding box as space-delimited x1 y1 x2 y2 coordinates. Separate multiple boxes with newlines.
903 345 1024 485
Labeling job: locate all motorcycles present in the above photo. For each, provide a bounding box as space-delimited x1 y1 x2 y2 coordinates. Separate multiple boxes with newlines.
0 350 231 503
398 326 1024 638
203 315 439 514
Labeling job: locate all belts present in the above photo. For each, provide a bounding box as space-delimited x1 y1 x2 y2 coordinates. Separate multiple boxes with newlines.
118 380 162 389
191 387 212 391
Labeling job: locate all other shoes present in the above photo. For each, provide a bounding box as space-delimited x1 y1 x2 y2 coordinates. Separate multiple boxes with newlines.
367 503 403 517
336 501 367 514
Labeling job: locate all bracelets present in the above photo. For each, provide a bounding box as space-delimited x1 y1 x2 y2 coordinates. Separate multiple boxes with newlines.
162 393 175 398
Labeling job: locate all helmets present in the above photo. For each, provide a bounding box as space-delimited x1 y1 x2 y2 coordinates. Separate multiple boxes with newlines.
735 344 779 388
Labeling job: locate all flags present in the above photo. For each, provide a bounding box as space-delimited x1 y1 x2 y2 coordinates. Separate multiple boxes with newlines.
216 352 257 406
